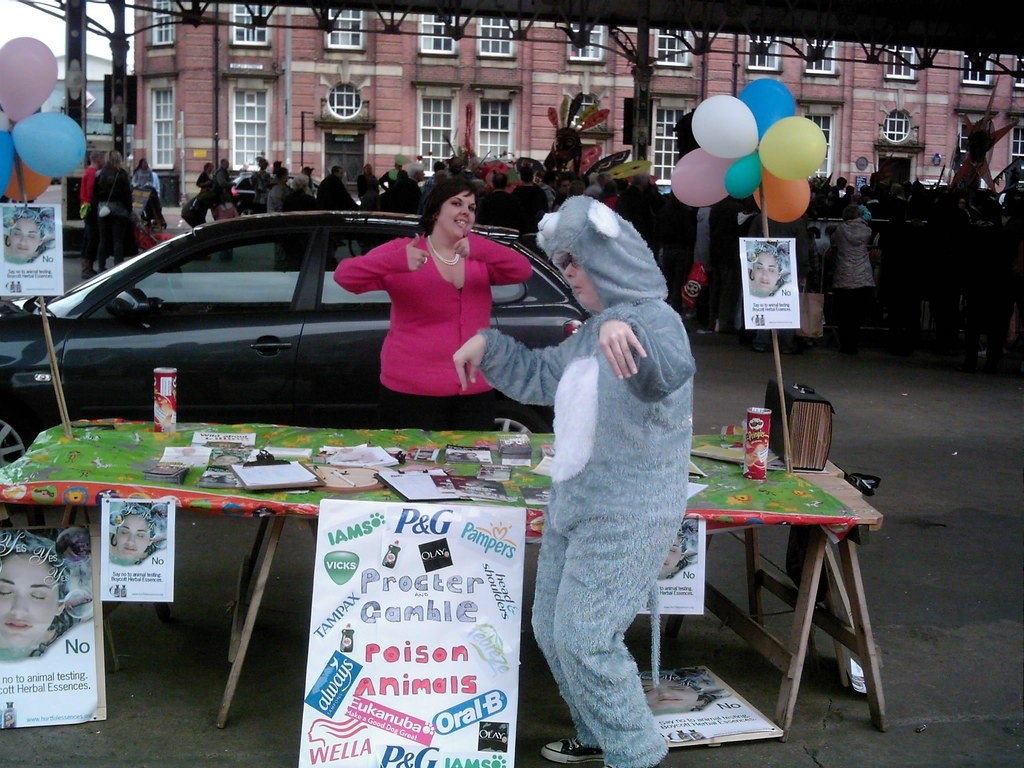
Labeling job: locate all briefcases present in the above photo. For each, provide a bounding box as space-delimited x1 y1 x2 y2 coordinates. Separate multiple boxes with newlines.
765 378 837 471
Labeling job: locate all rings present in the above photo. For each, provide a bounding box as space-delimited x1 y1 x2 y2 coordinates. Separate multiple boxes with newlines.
423 257 427 264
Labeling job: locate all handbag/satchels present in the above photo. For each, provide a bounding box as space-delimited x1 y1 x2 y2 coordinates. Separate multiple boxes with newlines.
182 198 203 228
796 287 825 338
97 201 129 219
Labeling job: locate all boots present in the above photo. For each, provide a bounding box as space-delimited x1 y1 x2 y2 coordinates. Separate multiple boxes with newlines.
80 257 98 279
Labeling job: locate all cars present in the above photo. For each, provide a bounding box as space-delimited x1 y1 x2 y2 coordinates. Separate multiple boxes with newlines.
231 174 322 215
356 171 437 207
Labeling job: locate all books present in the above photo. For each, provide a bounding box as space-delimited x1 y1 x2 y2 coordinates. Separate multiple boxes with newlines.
159 430 560 500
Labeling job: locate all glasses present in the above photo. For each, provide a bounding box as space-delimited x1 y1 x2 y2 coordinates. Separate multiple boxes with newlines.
562 254 579 270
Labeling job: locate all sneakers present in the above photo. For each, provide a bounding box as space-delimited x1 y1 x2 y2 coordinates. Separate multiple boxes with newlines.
541 736 604 763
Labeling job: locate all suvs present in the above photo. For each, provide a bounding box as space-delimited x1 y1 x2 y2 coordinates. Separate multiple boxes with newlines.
0 209 589 468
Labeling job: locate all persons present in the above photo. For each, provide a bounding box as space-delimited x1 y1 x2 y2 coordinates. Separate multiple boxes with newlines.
109 506 155 566
3 209 45 263
641 673 717 715
461 452 478 460
658 532 687 579
658 164 1024 374
0 530 65 653
749 243 786 298
454 195 695 768
333 178 532 432
175 149 659 257
447 451 467 460
81 150 160 280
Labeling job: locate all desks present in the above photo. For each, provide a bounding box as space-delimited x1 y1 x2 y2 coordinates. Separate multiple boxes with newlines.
0 418 888 740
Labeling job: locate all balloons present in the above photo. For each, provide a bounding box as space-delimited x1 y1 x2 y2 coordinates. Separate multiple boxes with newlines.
0 37 87 202
669 78 826 222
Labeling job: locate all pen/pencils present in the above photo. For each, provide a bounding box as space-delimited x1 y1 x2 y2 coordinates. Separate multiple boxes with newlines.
414 449 421 460
313 465 326 479
330 470 356 486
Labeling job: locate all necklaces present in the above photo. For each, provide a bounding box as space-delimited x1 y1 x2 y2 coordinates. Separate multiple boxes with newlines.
428 235 460 266
